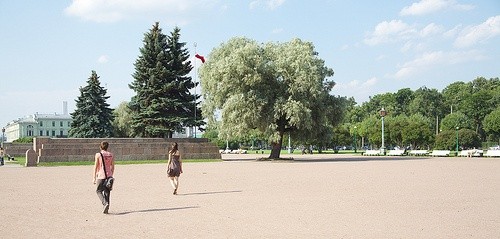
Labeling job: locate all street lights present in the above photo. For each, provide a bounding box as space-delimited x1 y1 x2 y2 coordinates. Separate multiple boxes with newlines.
455 125 460 157
379 108 387 156
354 126 357 153
2 127 6 148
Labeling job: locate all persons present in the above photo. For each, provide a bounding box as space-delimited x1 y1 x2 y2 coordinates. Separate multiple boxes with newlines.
0 148 4 165
92 141 114 214
167 142 183 194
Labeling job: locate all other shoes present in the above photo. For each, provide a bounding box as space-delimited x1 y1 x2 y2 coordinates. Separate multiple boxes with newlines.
173 189 177 194
102 204 109 214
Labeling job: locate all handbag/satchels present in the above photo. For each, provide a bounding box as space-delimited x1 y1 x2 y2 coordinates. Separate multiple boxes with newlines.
103 176 115 192
167 168 173 176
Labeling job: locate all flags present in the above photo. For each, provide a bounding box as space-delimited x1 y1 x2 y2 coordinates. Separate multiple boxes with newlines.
195 52 205 63
